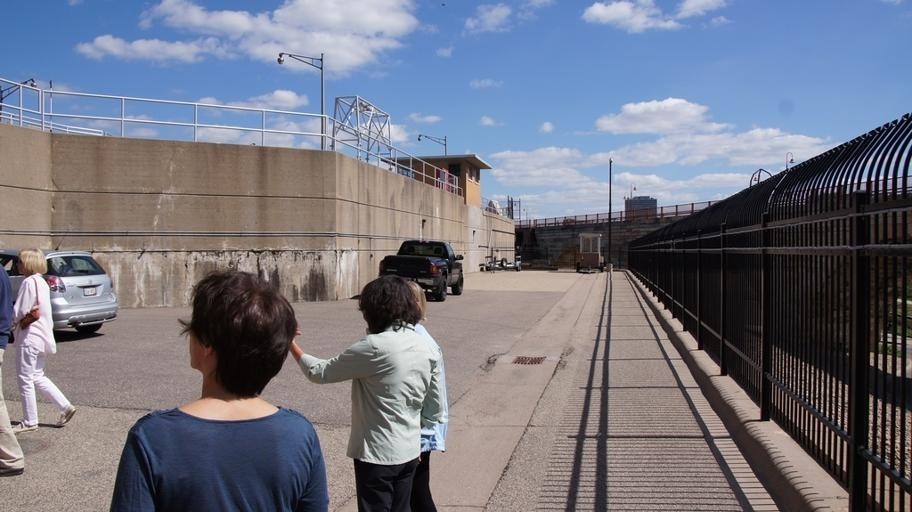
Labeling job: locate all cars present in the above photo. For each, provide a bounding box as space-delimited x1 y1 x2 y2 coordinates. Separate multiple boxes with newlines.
0 250 117 336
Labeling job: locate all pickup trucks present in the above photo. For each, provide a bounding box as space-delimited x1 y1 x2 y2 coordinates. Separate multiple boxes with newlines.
380 241 463 301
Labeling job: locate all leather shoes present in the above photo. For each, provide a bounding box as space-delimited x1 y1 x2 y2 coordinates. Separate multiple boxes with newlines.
0 467 24 477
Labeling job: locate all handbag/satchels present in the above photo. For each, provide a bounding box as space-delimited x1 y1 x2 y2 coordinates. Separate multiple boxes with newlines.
19 277 40 330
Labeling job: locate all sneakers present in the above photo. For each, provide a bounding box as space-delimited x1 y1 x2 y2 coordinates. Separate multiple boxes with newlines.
56 405 76 427
13 421 39 433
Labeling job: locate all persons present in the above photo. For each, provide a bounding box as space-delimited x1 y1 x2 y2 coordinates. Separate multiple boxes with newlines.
291 275 429 511
10 249 76 432
405 281 449 512
0 263 26 477
110 270 329 511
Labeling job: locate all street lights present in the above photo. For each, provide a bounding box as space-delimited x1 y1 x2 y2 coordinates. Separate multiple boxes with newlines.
0 78 37 123
607 156 613 262
278 53 326 151
785 152 796 169
630 182 637 198
418 133 447 156
